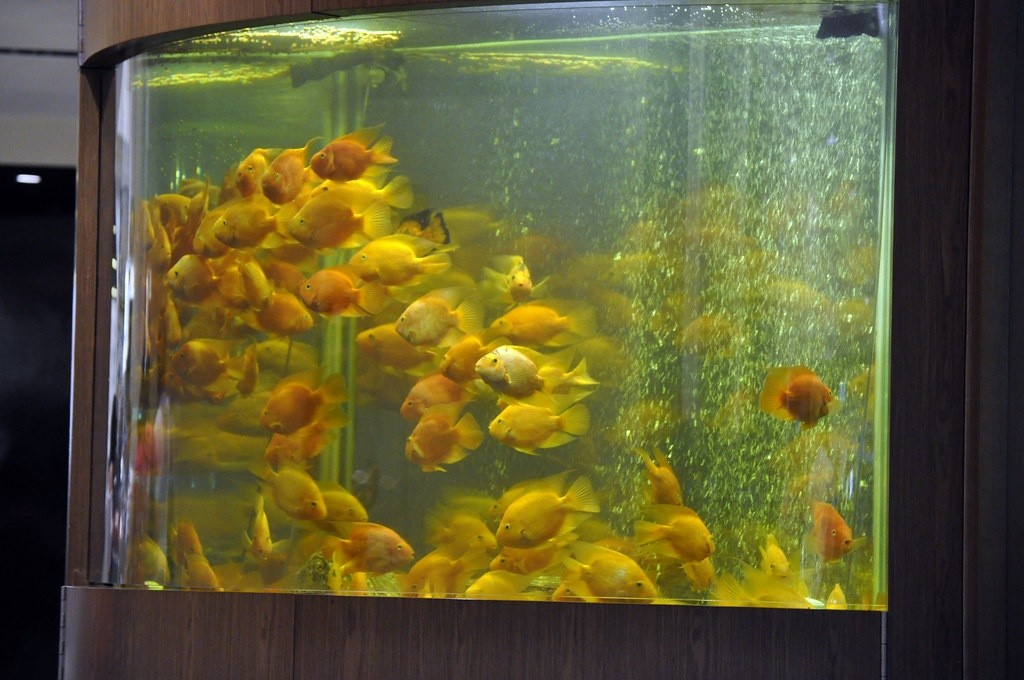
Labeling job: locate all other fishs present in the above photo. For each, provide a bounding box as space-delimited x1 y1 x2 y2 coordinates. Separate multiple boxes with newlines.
116 122 888 611
